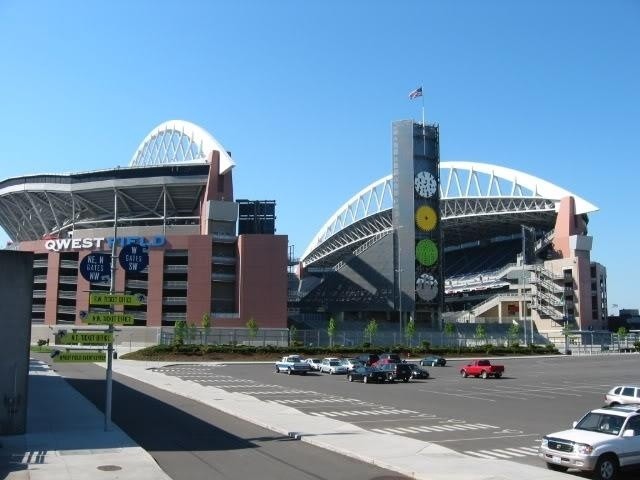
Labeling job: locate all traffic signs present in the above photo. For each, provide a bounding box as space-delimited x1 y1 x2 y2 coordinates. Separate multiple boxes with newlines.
50 292 143 363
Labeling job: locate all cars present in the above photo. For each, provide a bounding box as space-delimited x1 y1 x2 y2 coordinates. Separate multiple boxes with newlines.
274 352 429 384
419 354 446 367
539 386 640 480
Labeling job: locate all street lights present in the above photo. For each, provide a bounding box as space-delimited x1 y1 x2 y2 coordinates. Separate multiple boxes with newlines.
394 268 405 346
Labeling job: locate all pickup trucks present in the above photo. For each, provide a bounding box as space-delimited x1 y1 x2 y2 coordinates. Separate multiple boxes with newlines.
458 358 505 379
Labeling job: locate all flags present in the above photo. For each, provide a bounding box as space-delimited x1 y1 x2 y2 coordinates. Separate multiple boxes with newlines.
408 86 422 100
